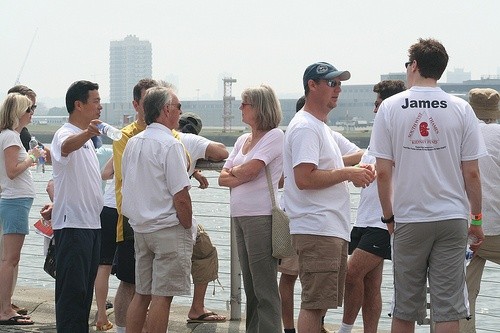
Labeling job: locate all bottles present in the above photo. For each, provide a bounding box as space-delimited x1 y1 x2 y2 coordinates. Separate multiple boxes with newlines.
37 146 45 177
95 120 122 141
29 137 38 169
465 233 480 267
352 146 376 187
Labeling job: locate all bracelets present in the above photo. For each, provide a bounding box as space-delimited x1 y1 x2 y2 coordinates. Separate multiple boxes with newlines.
231 167 235 177
471 214 482 221
470 220 483 225
29 155 36 163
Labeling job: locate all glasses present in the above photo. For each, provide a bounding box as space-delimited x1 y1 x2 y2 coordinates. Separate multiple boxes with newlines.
166 102 182 111
241 102 251 107
405 60 413 69
31 105 37 111
314 79 342 88
25 107 31 113
375 101 381 108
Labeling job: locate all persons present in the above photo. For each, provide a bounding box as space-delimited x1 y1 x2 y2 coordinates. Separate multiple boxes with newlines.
369 38 485 333
1 62 500 333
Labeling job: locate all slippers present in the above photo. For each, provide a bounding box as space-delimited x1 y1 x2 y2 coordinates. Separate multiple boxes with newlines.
12 304 28 315
187 312 226 322
0 314 35 325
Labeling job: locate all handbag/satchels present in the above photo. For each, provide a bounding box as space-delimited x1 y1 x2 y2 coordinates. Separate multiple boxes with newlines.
43 236 56 278
271 207 297 259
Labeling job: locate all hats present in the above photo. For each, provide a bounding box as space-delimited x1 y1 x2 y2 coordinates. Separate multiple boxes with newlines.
467 87 500 119
179 112 202 134
304 61 350 88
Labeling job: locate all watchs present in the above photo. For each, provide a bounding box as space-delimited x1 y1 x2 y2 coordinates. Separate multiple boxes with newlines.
381 215 394 223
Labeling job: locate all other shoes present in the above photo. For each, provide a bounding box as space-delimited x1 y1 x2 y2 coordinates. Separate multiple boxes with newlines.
90 320 117 333
